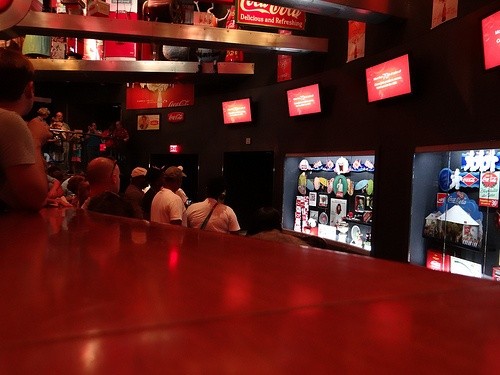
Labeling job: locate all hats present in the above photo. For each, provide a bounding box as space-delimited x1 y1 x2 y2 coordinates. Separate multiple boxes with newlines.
131 167 147 178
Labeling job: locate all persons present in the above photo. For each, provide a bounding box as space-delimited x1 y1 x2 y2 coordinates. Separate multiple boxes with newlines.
336 204 342 214
0 48 52 216
37 108 242 236
335 178 343 198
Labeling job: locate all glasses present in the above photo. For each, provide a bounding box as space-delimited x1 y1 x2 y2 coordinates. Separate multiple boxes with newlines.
107 155 118 179
